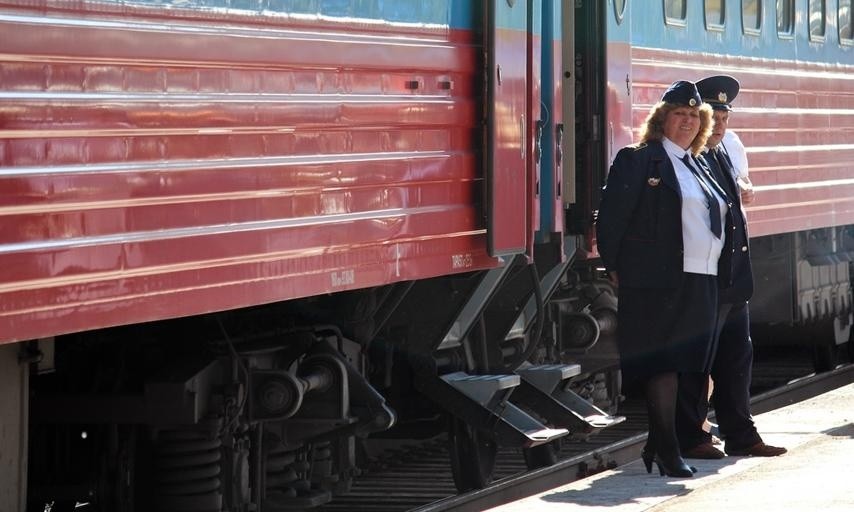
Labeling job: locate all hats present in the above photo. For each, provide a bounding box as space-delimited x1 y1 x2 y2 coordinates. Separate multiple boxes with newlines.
661 80 703 108
695 74 740 113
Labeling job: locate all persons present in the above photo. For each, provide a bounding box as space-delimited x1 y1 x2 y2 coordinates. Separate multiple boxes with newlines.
716 127 756 208
673 76 788 459
592 81 734 479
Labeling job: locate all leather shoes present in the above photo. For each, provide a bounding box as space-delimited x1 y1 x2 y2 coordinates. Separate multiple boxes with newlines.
680 442 724 460
724 441 787 457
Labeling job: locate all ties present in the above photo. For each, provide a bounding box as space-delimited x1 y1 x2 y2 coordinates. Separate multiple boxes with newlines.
681 153 722 240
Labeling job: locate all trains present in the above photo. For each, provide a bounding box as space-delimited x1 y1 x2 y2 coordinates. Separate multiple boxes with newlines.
0 0 854 512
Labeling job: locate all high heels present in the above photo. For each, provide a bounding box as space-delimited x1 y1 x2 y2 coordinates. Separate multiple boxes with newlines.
641 445 698 478
